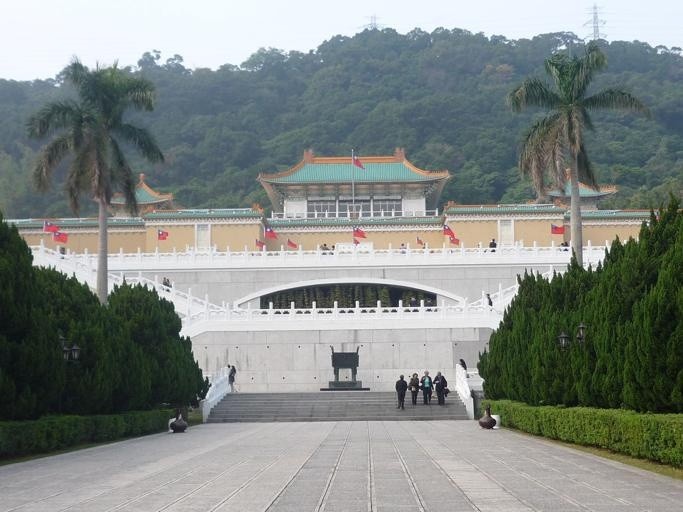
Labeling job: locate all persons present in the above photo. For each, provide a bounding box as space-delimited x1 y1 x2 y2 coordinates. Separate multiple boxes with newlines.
564 242 569 251
399 243 406 254
489 239 497 252
560 243 564 251
395 371 447 410
330 245 335 255
459 359 467 370
322 244 329 255
229 365 239 393
486 294 492 306
163 277 171 291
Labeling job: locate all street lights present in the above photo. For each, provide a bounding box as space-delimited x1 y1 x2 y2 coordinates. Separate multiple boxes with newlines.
56 333 81 415
557 322 587 355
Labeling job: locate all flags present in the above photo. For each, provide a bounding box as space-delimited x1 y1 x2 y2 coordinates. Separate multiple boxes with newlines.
353 226 367 238
43 220 60 232
265 226 279 240
287 239 297 249
158 230 168 240
353 238 360 245
352 151 365 169
416 237 424 245
551 224 564 234
52 231 67 243
450 237 459 245
443 224 455 238
255 239 264 248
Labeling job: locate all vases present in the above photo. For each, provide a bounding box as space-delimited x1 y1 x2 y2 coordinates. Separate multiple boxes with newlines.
490 413 500 427
478 403 496 429
170 407 187 432
168 418 176 432
